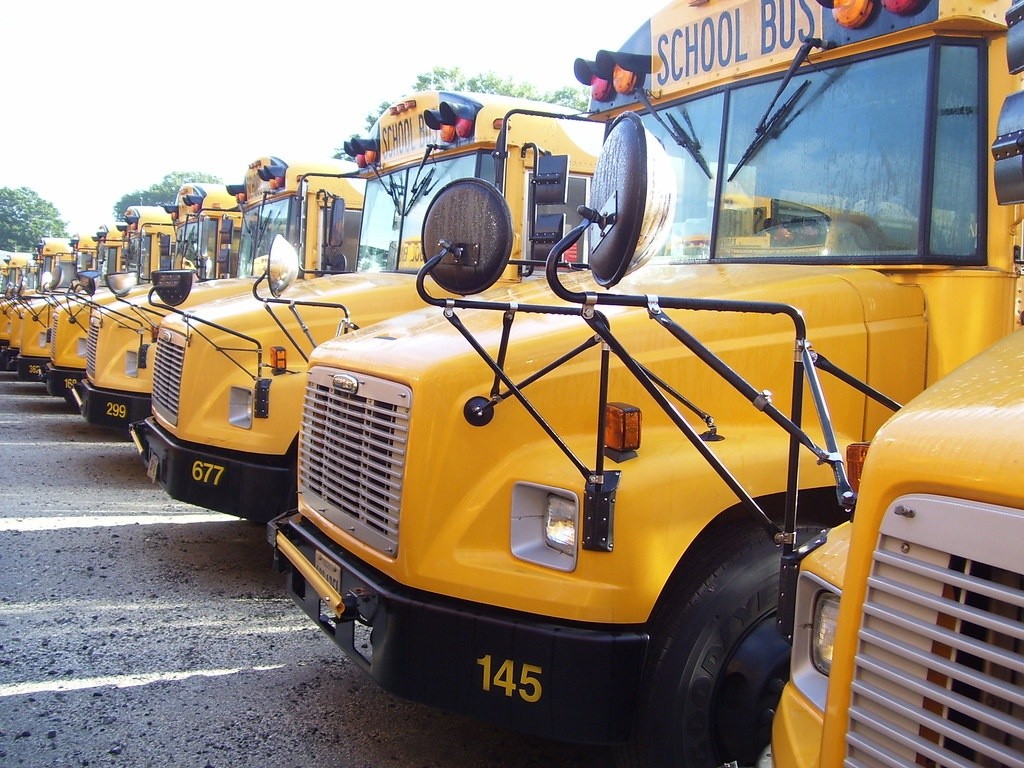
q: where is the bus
[66,155,366,430]
[104,90,605,527]
[714,195,889,256]
[253,0,1024,768]
[546,1,1024,768]
[0,206,175,399]
